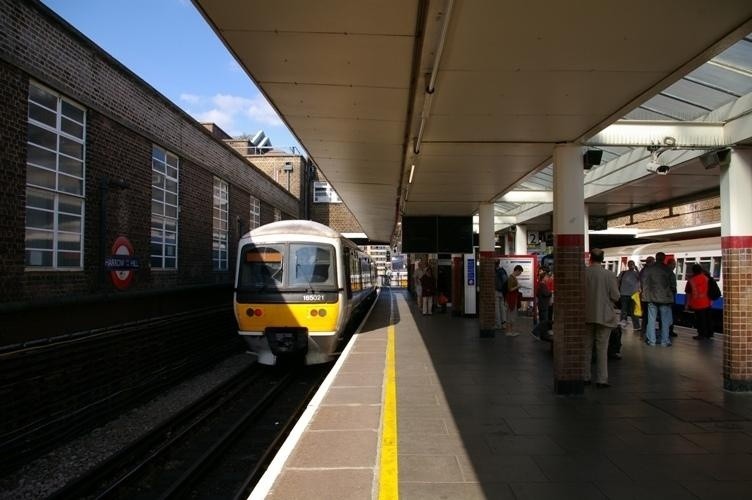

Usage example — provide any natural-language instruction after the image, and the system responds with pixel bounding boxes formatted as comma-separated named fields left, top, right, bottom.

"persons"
left=531, top=267, right=554, bottom=341
left=617, top=252, right=714, bottom=347
left=495, top=259, right=523, bottom=337
left=584, top=248, right=621, bottom=388
left=414, top=260, right=436, bottom=316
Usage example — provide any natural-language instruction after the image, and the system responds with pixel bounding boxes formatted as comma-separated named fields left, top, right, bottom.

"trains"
left=231, top=215, right=378, bottom=368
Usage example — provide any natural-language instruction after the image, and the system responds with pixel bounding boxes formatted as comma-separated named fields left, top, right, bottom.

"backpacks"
left=707, top=277, right=721, bottom=301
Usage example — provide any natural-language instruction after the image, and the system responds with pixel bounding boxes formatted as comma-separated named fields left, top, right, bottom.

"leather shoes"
left=633, top=327, right=677, bottom=346
left=585, top=380, right=609, bottom=390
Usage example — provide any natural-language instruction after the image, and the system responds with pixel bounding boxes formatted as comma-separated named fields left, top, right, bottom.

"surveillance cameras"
left=646, top=162, right=670, bottom=176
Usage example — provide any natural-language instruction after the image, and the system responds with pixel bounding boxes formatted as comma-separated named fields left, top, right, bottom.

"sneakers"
left=528, top=326, right=553, bottom=342
left=505, top=331, right=518, bottom=337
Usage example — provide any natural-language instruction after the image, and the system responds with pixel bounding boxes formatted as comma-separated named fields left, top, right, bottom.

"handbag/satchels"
left=631, top=292, right=644, bottom=317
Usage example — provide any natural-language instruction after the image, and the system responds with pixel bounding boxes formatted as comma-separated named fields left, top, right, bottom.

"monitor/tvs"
left=437, top=215, right=473, bottom=253
left=401, top=215, right=438, bottom=253
